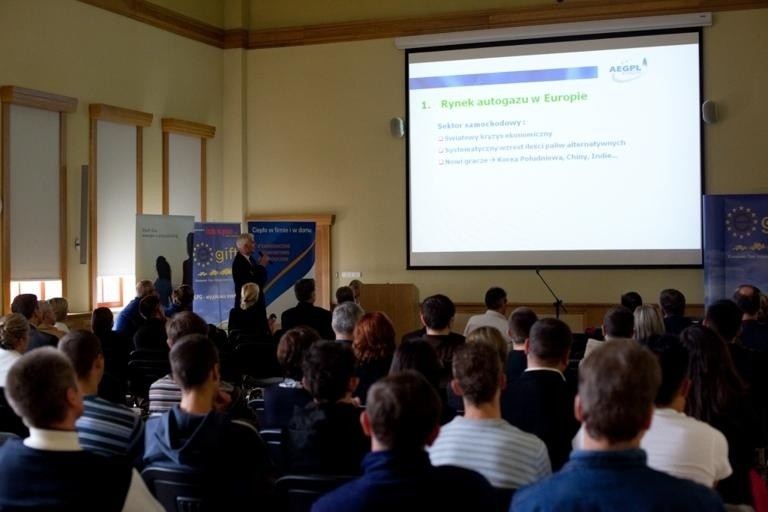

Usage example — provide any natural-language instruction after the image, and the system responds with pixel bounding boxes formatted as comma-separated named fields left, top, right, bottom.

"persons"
left=1, top=278, right=767, bottom=512
left=231, top=232, right=274, bottom=306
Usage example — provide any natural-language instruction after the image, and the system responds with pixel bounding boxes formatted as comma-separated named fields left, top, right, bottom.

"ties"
left=249, top=256, right=255, bottom=265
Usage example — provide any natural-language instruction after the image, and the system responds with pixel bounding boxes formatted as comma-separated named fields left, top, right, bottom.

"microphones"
left=257, top=248, right=271, bottom=263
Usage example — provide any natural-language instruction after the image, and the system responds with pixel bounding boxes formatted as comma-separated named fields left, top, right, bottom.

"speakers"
left=703, top=100, right=718, bottom=124
left=389, top=116, right=406, bottom=139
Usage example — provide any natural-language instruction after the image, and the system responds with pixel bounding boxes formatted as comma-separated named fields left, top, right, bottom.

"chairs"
left=141, top=465, right=362, bottom=511
left=125, top=351, right=169, bottom=404
